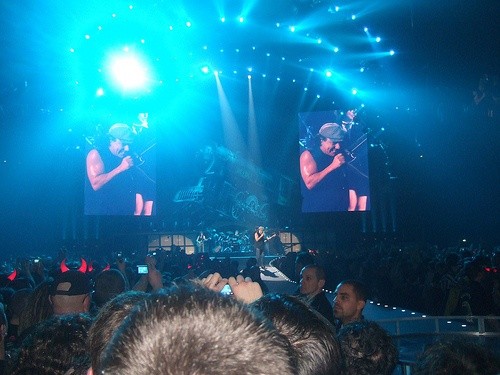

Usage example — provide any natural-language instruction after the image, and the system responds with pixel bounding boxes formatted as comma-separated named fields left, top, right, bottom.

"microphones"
left=335, top=149, right=347, bottom=176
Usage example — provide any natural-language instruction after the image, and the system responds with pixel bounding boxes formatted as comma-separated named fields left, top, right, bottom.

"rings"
left=231, top=282, right=239, bottom=289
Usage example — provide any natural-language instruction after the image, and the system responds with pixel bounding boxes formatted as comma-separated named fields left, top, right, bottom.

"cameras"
left=220, top=283, right=233, bottom=296
left=136, top=265, right=149, bottom=275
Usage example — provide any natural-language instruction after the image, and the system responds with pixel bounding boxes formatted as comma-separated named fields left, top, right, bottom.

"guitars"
left=196, top=237, right=211, bottom=247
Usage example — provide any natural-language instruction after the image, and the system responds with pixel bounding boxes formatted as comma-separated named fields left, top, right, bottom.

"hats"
left=319, top=122, right=345, bottom=142
left=49, top=271, right=91, bottom=296
left=109, top=123, right=134, bottom=145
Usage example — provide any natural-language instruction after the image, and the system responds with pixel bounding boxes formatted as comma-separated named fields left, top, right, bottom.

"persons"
left=0, top=245, right=500, bottom=375
left=83, top=112, right=155, bottom=217
left=255, top=226, right=270, bottom=270
left=299, top=122, right=345, bottom=211
left=345, top=109, right=370, bottom=212
left=196, top=231, right=208, bottom=252
